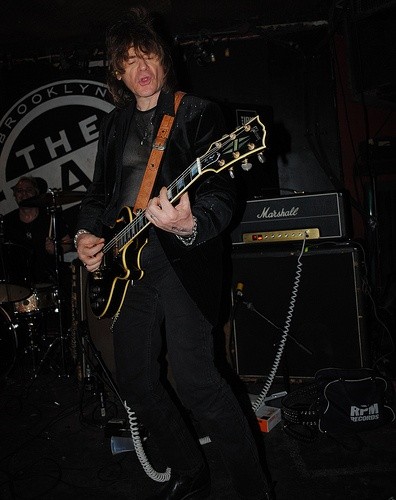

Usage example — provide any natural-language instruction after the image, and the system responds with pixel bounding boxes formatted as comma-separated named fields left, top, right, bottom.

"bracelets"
left=176, top=216, right=198, bottom=246
left=74, top=229, right=90, bottom=249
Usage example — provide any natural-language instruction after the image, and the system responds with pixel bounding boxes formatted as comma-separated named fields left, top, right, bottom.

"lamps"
left=180, top=35, right=217, bottom=71
left=76, top=27, right=106, bottom=63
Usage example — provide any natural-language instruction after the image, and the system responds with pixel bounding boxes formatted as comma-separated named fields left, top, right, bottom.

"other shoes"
left=145, top=463, right=211, bottom=500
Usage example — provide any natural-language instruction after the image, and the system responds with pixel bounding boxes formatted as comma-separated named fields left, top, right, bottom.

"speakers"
left=225, top=247, right=364, bottom=385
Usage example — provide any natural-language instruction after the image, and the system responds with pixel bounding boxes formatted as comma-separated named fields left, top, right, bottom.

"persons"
left=69, top=7, right=272, bottom=500
left=0, top=176, right=62, bottom=378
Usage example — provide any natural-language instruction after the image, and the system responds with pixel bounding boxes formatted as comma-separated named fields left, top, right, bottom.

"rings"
left=83, top=264, right=87, bottom=270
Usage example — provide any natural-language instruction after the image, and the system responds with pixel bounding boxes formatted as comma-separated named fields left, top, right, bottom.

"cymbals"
left=19, top=189, right=87, bottom=209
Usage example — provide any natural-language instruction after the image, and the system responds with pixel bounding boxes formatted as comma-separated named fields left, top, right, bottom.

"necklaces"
left=134, top=108, right=155, bottom=146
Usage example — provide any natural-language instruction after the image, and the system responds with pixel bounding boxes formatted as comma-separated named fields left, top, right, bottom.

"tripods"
left=30, top=204, right=84, bottom=382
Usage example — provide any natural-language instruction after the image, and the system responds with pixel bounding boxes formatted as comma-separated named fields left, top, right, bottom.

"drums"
left=0, top=241, right=33, bottom=303
left=0, top=305, right=28, bottom=384
left=30, top=280, right=61, bottom=308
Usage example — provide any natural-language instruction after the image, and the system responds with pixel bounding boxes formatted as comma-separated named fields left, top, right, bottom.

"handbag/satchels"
left=281, top=365, right=396, bottom=433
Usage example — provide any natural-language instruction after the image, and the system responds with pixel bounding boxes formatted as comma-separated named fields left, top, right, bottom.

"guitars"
left=86, top=115, right=268, bottom=320
